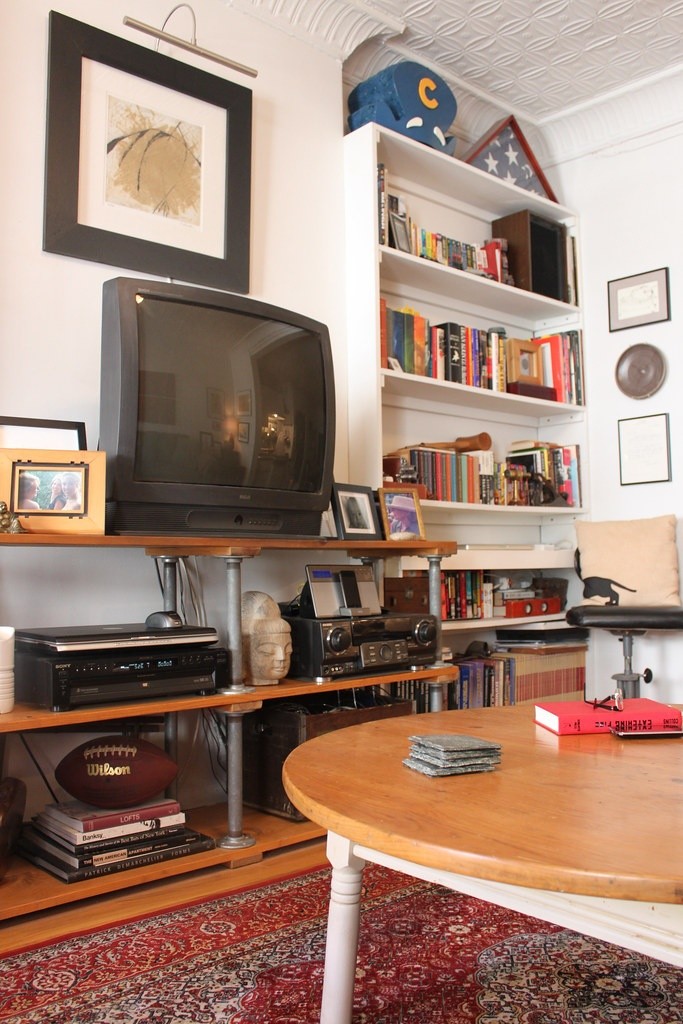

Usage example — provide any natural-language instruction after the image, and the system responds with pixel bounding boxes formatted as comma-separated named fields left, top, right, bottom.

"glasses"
left=584, top=682, right=623, bottom=712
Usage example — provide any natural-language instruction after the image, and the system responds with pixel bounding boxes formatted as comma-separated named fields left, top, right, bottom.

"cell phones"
left=338, top=570, right=362, bottom=609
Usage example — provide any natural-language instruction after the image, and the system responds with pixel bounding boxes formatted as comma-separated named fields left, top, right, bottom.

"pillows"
left=575, top=514, right=683, bottom=606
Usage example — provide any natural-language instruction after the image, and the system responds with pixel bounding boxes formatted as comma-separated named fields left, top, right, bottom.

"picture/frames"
left=238, top=422, right=249, bottom=443
left=330, top=483, right=383, bottom=540
left=390, top=211, right=412, bottom=254
left=0, top=448, right=106, bottom=535
left=213, top=442, right=224, bottom=460
left=236, top=389, right=252, bottom=417
left=199, top=432, right=214, bottom=449
left=43, top=11, right=252, bottom=295
left=617, top=414, right=673, bottom=485
left=206, top=387, right=226, bottom=420
left=607, top=267, right=671, bottom=332
left=378, top=488, right=427, bottom=541
left=0, top=416, right=87, bottom=451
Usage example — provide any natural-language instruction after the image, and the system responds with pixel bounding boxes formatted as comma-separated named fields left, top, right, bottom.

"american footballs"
left=54, top=735, right=179, bottom=810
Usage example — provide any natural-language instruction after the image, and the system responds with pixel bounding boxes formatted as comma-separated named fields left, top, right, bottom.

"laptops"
left=15, top=623, right=219, bottom=659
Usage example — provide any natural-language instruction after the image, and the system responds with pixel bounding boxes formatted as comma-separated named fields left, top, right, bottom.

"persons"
left=387, top=496, right=420, bottom=538
left=346, top=497, right=369, bottom=529
left=18, top=473, right=82, bottom=511
left=239, top=591, right=293, bottom=687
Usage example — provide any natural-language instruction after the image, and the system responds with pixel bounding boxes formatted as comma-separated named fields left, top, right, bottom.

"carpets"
left=0, top=860, right=683, bottom=1024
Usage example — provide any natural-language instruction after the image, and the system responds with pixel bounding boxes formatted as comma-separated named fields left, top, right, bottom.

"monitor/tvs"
left=96, top=276, right=335, bottom=537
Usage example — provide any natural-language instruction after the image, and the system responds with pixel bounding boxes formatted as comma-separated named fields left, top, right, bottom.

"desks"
left=283, top=703, right=683, bottom=1024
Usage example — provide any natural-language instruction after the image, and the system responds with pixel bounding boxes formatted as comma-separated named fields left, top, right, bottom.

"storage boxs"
left=492, top=210, right=571, bottom=303
left=506, top=338, right=557, bottom=401
left=226, top=695, right=417, bottom=820
left=383, top=577, right=429, bottom=615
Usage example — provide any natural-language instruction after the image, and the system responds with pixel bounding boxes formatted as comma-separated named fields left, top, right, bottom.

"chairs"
left=566, top=547, right=683, bottom=699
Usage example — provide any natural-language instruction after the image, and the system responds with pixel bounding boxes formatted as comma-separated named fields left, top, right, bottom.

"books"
left=378, top=163, right=516, bottom=287
left=379, top=298, right=584, bottom=408
left=402, top=568, right=493, bottom=618
left=381, top=440, right=582, bottom=509
left=17, top=798, right=216, bottom=886
left=532, top=698, right=683, bottom=737
left=373, top=646, right=587, bottom=717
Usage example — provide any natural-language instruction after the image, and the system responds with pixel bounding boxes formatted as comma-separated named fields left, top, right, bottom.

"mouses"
left=146, top=611, right=183, bottom=628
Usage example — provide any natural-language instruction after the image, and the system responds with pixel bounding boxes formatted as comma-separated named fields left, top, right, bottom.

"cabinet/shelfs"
left=344, top=122, right=591, bottom=631
left=0, top=532, right=458, bottom=919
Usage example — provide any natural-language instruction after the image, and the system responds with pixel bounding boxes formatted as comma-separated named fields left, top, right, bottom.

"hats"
left=385, top=496, right=416, bottom=513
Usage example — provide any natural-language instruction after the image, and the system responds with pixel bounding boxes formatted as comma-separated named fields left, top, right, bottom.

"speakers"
left=493, top=210, right=571, bottom=304
